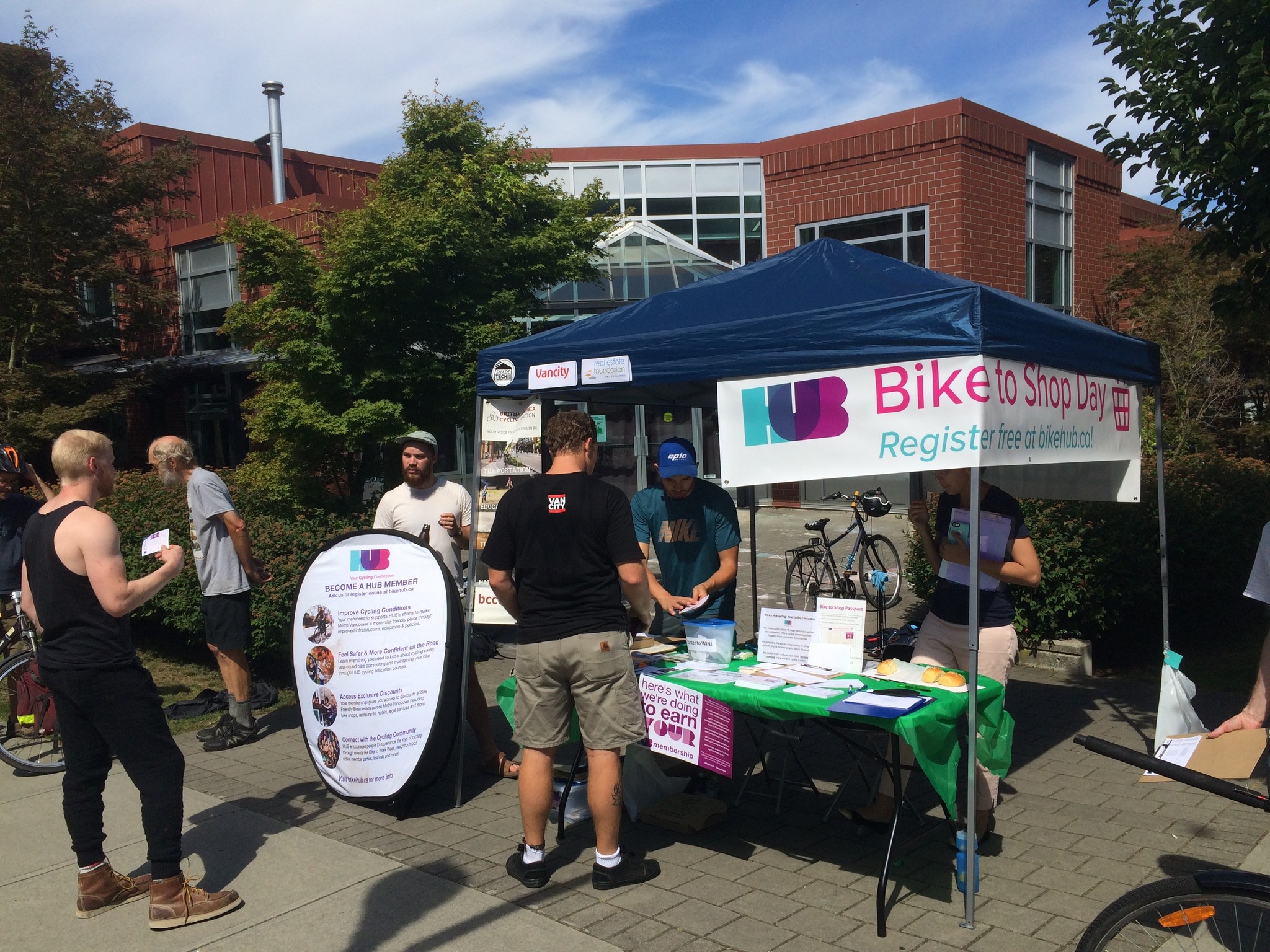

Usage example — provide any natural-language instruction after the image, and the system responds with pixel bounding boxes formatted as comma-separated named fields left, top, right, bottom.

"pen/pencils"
left=848, top=684, right=852, bottom=695
left=854, top=684, right=867, bottom=692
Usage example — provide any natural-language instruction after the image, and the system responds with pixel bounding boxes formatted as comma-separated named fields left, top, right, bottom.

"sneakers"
left=505, top=844, right=548, bottom=887
left=196, top=712, right=236, bottom=740
left=203, top=717, right=259, bottom=751
left=593, top=853, right=660, bottom=890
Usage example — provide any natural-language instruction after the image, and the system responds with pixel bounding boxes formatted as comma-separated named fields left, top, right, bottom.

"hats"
left=395, top=430, right=438, bottom=452
left=657, top=436, right=698, bottom=478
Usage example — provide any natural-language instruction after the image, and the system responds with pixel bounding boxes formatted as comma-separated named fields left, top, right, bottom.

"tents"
left=445, top=234, right=1174, bottom=932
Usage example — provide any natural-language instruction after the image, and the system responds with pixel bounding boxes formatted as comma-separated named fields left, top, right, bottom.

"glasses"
left=0, top=477, right=19, bottom=484
left=150, top=460, right=164, bottom=472
left=933, top=470, right=949, bottom=475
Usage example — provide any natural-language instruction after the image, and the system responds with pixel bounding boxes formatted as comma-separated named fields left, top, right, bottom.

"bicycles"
left=785, top=488, right=903, bottom=613
left=1, top=589, right=67, bottom=776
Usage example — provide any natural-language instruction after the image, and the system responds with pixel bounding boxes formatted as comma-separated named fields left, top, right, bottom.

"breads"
left=876, top=660, right=898, bottom=675
left=937, top=671, right=966, bottom=688
left=921, top=667, right=946, bottom=683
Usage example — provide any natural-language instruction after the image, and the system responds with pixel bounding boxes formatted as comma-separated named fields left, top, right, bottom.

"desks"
left=496, top=635, right=1016, bottom=938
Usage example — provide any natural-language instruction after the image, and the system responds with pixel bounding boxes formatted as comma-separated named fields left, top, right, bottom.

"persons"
left=0, top=442, right=56, bottom=740
left=832, top=468, right=1042, bottom=850
left=312, top=695, right=337, bottom=726
left=478, top=409, right=663, bottom=891
left=1206, top=514, right=1269, bottom=739
left=147, top=436, right=275, bottom=753
left=482, top=485, right=488, bottom=503
left=313, top=607, right=327, bottom=635
left=19, top=429, right=243, bottom=930
left=307, top=647, right=334, bottom=685
left=370, top=430, right=521, bottom=779
left=503, top=452, right=510, bottom=468
left=629, top=437, right=742, bottom=796
left=506, top=477, right=514, bottom=488
left=317, top=734, right=340, bottom=768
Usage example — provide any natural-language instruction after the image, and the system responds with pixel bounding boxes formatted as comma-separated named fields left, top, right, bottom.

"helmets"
left=863, top=496, right=892, bottom=517
left=1, top=442, right=28, bottom=473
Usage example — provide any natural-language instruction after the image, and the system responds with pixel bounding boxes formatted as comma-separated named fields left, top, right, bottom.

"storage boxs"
left=681, top=616, right=741, bottom=665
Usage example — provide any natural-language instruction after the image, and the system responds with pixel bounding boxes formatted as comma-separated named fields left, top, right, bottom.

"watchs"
left=449, top=525, right=462, bottom=538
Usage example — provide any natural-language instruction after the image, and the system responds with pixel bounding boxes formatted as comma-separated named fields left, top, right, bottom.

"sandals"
left=479, top=751, right=520, bottom=777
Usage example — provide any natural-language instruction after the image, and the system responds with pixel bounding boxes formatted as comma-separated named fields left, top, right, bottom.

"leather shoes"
left=75, top=863, right=152, bottom=918
left=148, top=872, right=241, bottom=930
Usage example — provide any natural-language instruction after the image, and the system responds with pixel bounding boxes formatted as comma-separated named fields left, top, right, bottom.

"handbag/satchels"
left=15, top=661, right=61, bottom=734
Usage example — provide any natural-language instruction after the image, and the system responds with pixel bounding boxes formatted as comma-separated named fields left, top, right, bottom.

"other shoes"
left=978, top=825, right=990, bottom=845
left=835, top=802, right=896, bottom=835
